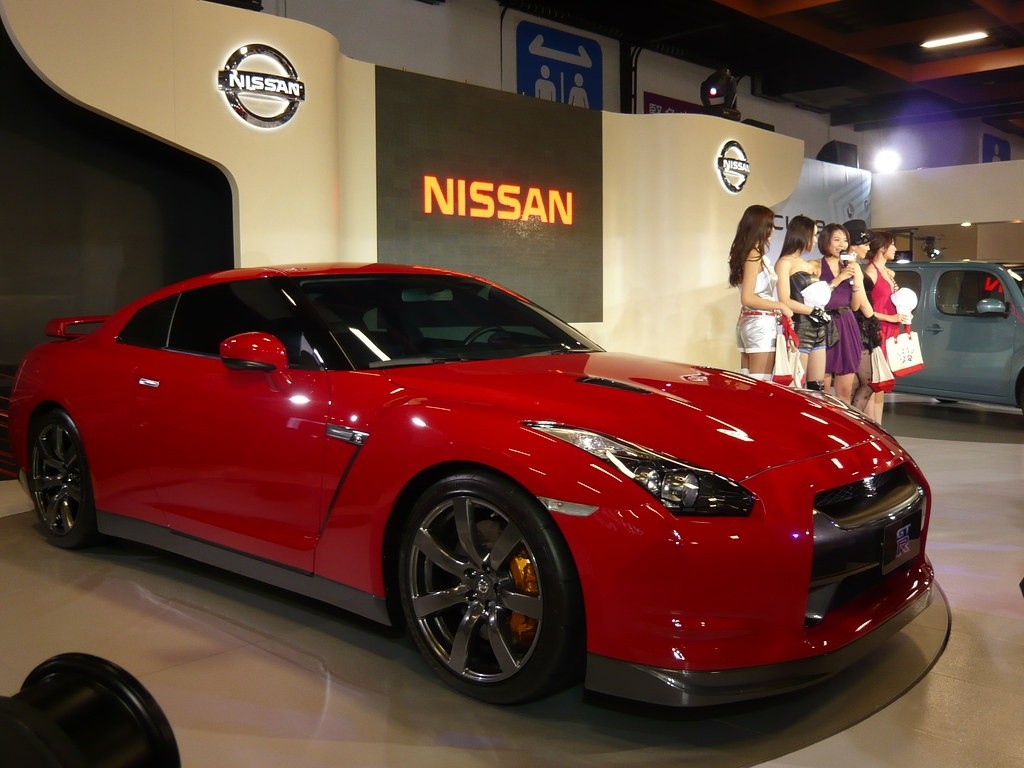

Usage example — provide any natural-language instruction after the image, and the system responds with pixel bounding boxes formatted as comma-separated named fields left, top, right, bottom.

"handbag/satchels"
left=772, top=315, right=807, bottom=390
left=883, top=323, right=924, bottom=378
left=867, top=345, right=897, bottom=394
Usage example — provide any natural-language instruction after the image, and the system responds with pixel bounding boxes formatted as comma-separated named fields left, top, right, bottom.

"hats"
left=801, top=280, right=831, bottom=311
left=843, top=219, right=874, bottom=246
left=890, top=288, right=917, bottom=325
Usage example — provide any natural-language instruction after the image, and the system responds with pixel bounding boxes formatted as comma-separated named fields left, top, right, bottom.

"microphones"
left=839, top=250, right=855, bottom=285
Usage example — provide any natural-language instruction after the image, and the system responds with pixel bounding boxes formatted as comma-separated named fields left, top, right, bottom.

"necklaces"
left=884, top=267, right=897, bottom=287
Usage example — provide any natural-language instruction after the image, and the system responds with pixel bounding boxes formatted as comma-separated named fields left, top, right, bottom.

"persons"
left=774, top=215, right=830, bottom=392
left=863, top=232, right=908, bottom=422
left=843, top=219, right=882, bottom=411
left=809, top=223, right=861, bottom=404
left=730, top=206, right=794, bottom=379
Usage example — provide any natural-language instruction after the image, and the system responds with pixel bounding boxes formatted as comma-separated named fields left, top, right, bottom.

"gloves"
left=810, top=306, right=833, bottom=328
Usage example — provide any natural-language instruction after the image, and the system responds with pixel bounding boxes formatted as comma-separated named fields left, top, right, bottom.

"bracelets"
left=829, top=282, right=836, bottom=289
left=851, top=289, right=861, bottom=293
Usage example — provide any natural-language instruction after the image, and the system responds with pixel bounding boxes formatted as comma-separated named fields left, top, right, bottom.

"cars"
left=10, top=263, right=934, bottom=710
left=804, top=263, right=1024, bottom=407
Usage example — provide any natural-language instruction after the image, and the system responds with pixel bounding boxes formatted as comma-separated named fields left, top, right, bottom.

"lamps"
left=700, top=71, right=742, bottom=121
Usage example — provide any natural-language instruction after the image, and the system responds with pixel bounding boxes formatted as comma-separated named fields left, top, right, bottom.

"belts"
left=741, top=312, right=775, bottom=316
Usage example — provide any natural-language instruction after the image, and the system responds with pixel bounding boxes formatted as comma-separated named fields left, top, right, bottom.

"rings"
left=791, top=314, right=793, bottom=316
